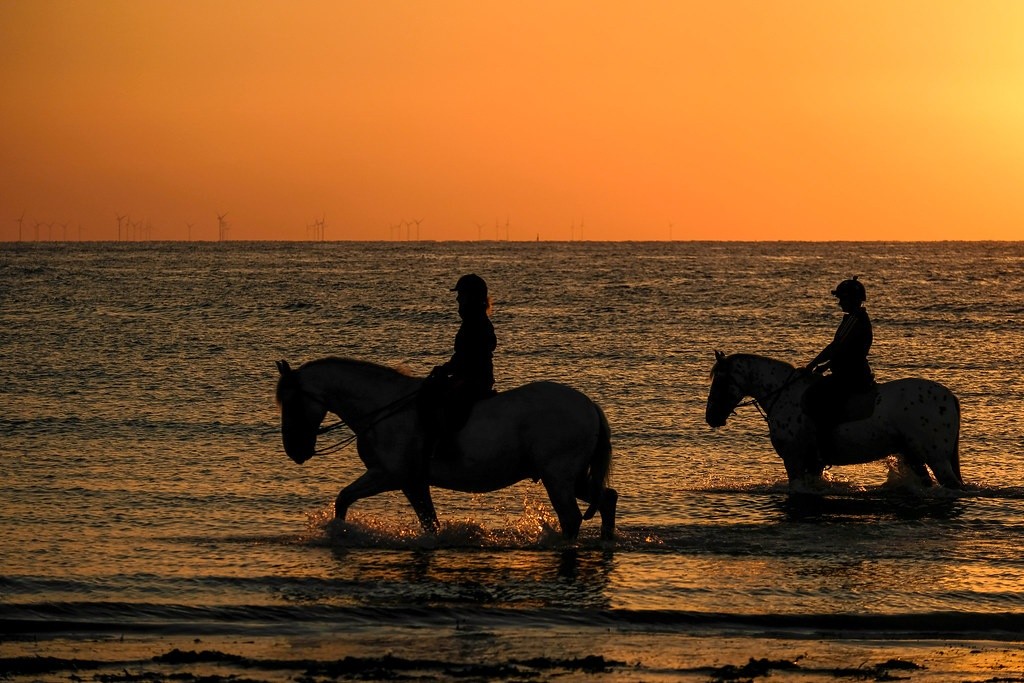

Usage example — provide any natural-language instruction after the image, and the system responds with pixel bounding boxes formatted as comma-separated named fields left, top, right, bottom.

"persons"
left=801, top=276, right=873, bottom=463
left=408, top=274, right=497, bottom=480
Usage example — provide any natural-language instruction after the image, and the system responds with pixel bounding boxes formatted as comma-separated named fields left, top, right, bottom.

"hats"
left=831, top=275, right=868, bottom=302
left=450, top=273, right=487, bottom=291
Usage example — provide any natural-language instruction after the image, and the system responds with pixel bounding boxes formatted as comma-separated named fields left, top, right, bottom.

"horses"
left=707, top=349, right=963, bottom=497
left=275, top=357, right=618, bottom=540
